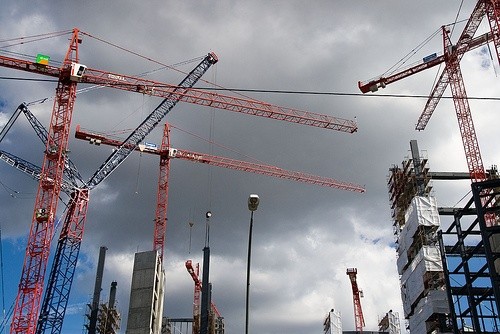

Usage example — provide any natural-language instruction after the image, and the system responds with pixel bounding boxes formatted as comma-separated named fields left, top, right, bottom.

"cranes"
left=0, top=25, right=365, bottom=333
left=359, top=1, right=499, bottom=227
left=415, top=0, right=500, bottom=132
